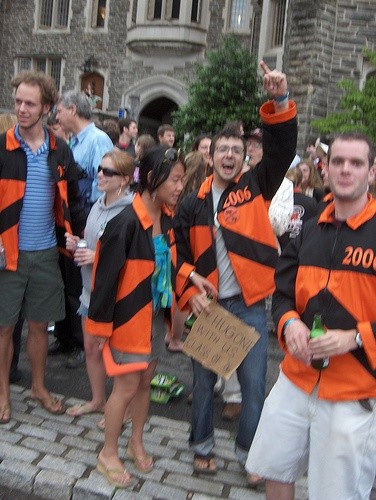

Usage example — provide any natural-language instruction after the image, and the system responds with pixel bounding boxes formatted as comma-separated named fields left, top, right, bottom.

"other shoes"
left=240, top=460, right=265, bottom=485
left=47, top=339, right=71, bottom=354
left=193, top=452, right=218, bottom=473
left=189, top=390, right=218, bottom=404
left=65, top=349, right=86, bottom=368
left=222, top=403, right=241, bottom=421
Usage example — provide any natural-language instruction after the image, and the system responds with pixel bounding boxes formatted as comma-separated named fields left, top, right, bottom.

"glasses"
left=97, top=165, right=126, bottom=177
left=218, top=145, right=245, bottom=157
left=163, top=148, right=185, bottom=164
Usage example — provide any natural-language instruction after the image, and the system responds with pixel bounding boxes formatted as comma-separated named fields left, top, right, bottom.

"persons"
left=84, top=144, right=219, bottom=488
left=244, top=129, right=376, bottom=500
left=64, top=150, right=134, bottom=430
left=172, top=58, right=298, bottom=490
left=0, top=60, right=376, bottom=500
left=0, top=70, right=73, bottom=426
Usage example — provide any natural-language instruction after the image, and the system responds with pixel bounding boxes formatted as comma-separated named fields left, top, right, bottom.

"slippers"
left=30, top=392, right=66, bottom=414
left=127, top=446, right=153, bottom=471
left=97, top=462, right=130, bottom=487
left=0, top=401, right=12, bottom=423
left=97, top=415, right=134, bottom=430
left=164, top=342, right=183, bottom=353
left=67, top=402, right=104, bottom=416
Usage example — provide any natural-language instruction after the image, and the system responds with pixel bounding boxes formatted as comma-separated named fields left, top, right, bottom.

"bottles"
left=184, top=294, right=213, bottom=329
left=310, top=313, right=329, bottom=369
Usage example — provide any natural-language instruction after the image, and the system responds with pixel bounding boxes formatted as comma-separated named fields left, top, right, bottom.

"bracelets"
left=189, top=271, right=196, bottom=278
left=274, top=98, right=289, bottom=107
left=273, top=92, right=288, bottom=101
left=283, top=318, right=296, bottom=328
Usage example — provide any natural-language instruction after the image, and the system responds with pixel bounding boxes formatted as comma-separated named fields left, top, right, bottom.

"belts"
left=360, top=398, right=374, bottom=412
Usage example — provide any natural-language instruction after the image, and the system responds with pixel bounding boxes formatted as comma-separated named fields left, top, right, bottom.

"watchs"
left=355, top=331, right=364, bottom=351
